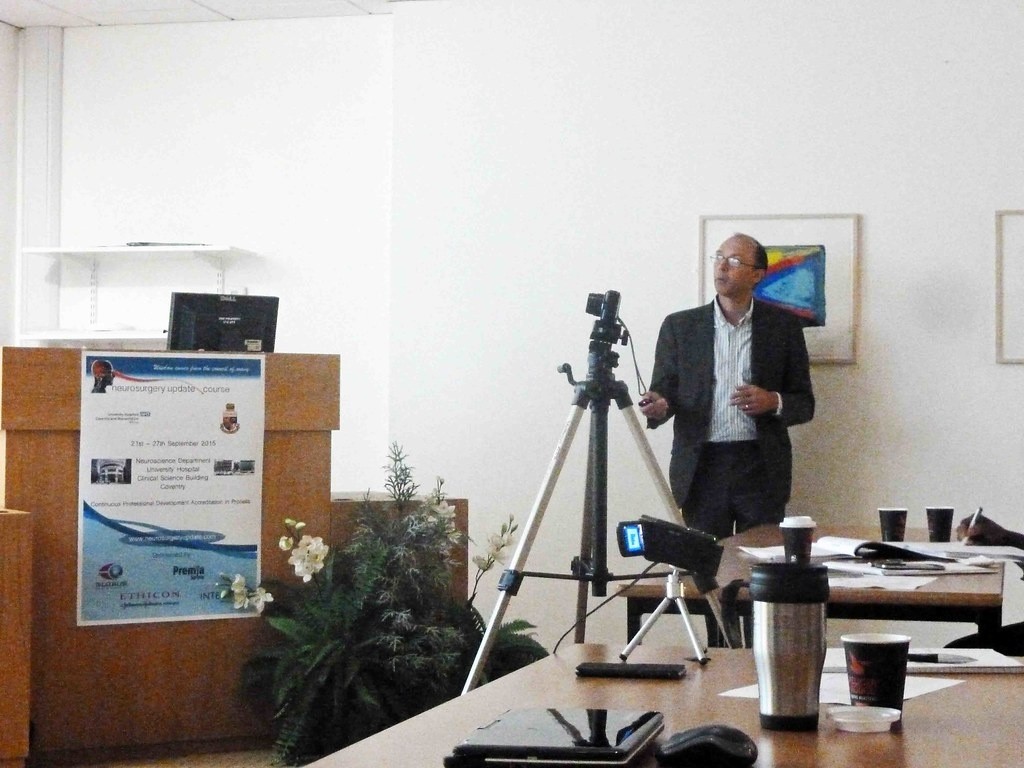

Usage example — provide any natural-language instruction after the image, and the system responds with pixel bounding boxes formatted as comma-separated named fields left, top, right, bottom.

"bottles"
left=222, top=404, right=238, bottom=430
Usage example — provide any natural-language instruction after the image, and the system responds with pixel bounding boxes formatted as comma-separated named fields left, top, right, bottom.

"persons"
left=943, top=513, right=1024, bottom=656
left=639, top=233, right=815, bottom=649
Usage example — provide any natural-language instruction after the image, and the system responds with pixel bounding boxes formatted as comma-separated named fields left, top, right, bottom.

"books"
left=823, top=647, right=1024, bottom=674
left=736, top=536, right=957, bottom=564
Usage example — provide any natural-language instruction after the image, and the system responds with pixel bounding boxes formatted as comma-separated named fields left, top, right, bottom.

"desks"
left=617, top=524, right=1005, bottom=648
left=297, top=640, right=1024, bottom=768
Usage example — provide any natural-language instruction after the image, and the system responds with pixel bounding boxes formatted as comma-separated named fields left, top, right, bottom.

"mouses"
left=655, top=725, right=758, bottom=768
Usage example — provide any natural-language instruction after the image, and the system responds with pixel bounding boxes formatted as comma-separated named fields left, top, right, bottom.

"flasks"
left=751, top=562, right=830, bottom=732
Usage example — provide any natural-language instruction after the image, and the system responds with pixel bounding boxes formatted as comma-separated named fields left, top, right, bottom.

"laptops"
left=443, top=709, right=665, bottom=768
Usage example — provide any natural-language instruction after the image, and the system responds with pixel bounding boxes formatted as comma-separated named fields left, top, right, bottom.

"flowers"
left=206, top=441, right=550, bottom=768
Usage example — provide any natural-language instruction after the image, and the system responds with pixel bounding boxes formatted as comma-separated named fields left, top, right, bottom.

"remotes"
left=638, top=398, right=653, bottom=407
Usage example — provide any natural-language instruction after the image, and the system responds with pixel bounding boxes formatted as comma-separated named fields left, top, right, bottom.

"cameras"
left=586, top=290, right=621, bottom=321
left=616, top=514, right=723, bottom=576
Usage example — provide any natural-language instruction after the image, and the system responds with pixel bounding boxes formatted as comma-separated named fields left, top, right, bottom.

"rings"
left=746, top=404, right=748, bottom=408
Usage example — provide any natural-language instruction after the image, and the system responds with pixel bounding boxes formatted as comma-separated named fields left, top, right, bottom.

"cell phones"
left=575, top=662, right=686, bottom=680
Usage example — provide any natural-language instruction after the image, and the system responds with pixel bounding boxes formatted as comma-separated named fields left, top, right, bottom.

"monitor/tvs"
left=167, top=292, right=279, bottom=353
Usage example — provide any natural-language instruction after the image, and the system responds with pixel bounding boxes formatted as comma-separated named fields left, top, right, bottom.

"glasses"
left=710, top=254, right=757, bottom=269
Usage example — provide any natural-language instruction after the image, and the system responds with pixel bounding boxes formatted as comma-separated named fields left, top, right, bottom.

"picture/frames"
left=993, top=210, right=1024, bottom=366
left=698, top=212, right=862, bottom=371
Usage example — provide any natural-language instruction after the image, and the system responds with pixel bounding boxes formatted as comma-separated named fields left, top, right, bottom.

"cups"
left=925, top=506, right=955, bottom=542
left=779, top=516, right=817, bottom=564
left=841, top=633, right=913, bottom=732
left=878, top=507, right=908, bottom=541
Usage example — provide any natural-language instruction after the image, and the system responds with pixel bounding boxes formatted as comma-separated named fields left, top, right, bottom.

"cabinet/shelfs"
left=21, top=246, right=248, bottom=340
left=330, top=490, right=470, bottom=602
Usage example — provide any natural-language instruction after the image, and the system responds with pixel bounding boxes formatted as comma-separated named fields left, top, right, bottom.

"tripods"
left=462, top=318, right=734, bottom=699
left=619, top=566, right=708, bottom=666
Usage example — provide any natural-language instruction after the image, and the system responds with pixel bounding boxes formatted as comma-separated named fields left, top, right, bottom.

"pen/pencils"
left=907, top=653, right=978, bottom=663
left=962, top=507, right=983, bottom=545
